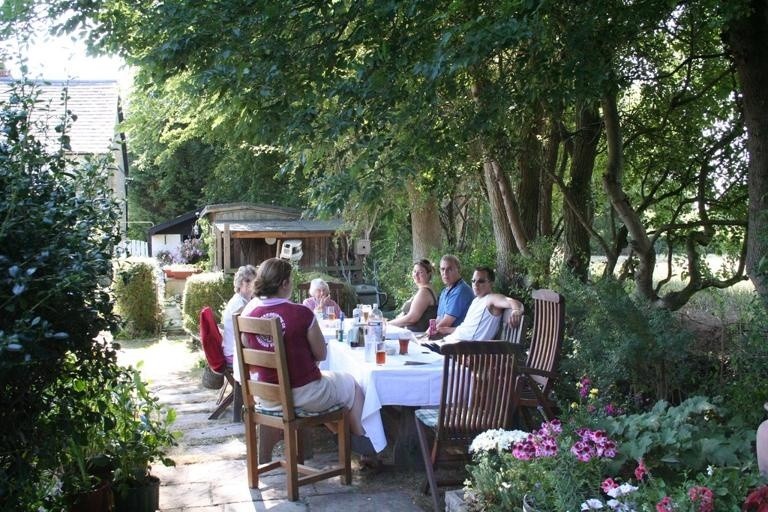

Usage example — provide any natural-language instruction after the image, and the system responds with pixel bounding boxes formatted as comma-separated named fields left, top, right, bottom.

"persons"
left=231, top=291, right=266, bottom=386
left=300, top=277, right=344, bottom=319
left=222, top=265, right=259, bottom=364
left=420, top=267, right=525, bottom=368
left=240, top=256, right=383, bottom=469
left=416, top=254, right=475, bottom=341
left=388, top=259, right=436, bottom=335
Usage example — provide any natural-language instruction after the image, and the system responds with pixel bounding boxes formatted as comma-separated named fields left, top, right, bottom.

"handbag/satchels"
left=202, top=364, right=224, bottom=389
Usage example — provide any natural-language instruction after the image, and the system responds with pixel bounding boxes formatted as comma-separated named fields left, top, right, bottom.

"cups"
left=375, top=342, right=386, bottom=366
left=398, top=335, right=410, bottom=355
left=327, top=306, right=335, bottom=324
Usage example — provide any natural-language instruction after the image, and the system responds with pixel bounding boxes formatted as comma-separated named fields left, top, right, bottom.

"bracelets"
left=511, top=309, right=520, bottom=313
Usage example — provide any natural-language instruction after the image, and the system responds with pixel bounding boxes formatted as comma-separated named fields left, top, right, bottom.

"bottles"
left=348, top=303, right=383, bottom=342
left=349, top=342, right=376, bottom=361
left=335, top=311, right=345, bottom=342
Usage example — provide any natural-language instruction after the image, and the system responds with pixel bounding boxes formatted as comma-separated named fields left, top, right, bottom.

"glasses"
left=471, top=278, right=489, bottom=283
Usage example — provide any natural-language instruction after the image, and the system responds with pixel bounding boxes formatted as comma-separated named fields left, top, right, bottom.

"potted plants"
left=30, top=351, right=187, bottom=512
